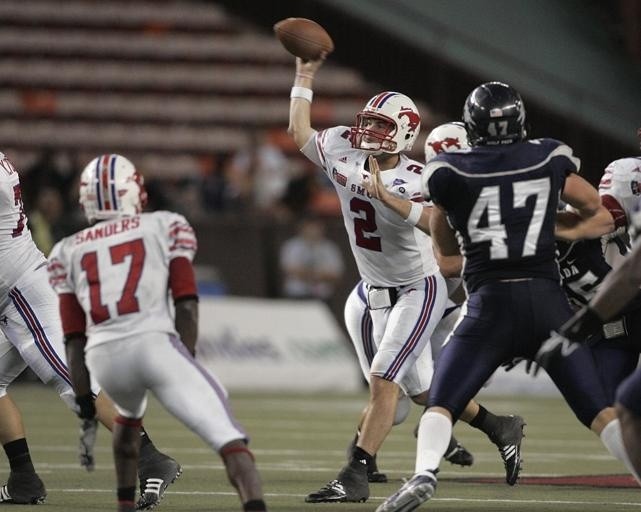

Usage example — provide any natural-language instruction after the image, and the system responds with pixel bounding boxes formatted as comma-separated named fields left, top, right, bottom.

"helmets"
left=349, top=82, right=528, bottom=163
left=76, top=154, right=149, bottom=224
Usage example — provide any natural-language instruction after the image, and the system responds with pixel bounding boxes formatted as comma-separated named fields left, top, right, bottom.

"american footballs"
left=274, top=16, right=334, bottom=61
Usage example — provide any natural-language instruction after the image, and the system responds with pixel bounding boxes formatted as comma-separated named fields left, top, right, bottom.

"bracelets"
left=295, top=70, right=316, bottom=81
left=287, top=86, right=316, bottom=104
left=73, top=392, right=97, bottom=420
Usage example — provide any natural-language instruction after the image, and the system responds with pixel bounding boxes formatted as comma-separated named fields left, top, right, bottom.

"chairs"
left=1, top=1, right=446, bottom=293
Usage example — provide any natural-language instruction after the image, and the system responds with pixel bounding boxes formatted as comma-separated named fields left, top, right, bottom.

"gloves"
left=525, top=306, right=603, bottom=378
left=79, top=418, right=98, bottom=472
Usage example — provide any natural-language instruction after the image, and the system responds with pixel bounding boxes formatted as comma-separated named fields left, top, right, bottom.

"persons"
left=282, top=50, right=527, bottom=503
left=44, top=154, right=269, bottom=511
left=341, top=79, right=640, bottom=511
left=26, top=120, right=346, bottom=302
left=1, top=152, right=185, bottom=512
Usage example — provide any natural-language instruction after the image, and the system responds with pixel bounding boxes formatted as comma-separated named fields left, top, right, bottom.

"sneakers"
left=133, top=446, right=183, bottom=511
left=0, top=473, right=47, bottom=505
left=489, top=414, right=526, bottom=486
left=413, top=425, right=473, bottom=466
left=304, top=441, right=440, bottom=512
